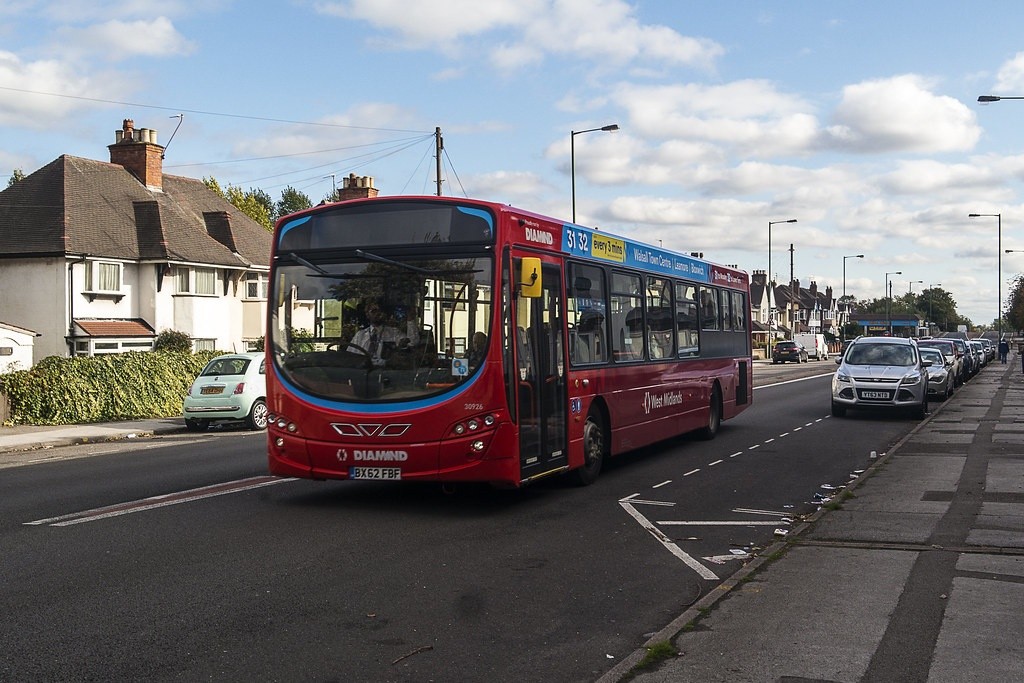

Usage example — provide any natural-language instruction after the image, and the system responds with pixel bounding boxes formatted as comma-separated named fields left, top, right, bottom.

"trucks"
left=794, top=332, right=829, bottom=361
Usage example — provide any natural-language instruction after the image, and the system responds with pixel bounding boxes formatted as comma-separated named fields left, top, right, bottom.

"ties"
left=367, top=328, right=377, bottom=357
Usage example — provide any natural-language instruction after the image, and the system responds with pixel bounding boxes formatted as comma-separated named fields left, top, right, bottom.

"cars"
left=918, top=347, right=955, bottom=401
left=182, top=351, right=285, bottom=433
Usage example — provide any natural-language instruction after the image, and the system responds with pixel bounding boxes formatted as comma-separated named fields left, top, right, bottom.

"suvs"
left=831, top=334, right=938, bottom=420
left=772, top=340, right=808, bottom=364
left=914, top=334, right=997, bottom=389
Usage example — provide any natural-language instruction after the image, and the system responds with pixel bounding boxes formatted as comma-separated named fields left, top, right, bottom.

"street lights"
left=929, top=284, right=942, bottom=335
left=909, top=280, right=923, bottom=338
left=886, top=271, right=902, bottom=331
left=969, top=214, right=1002, bottom=361
left=768, top=219, right=799, bottom=360
left=571, top=124, right=620, bottom=329
left=843, top=255, right=864, bottom=340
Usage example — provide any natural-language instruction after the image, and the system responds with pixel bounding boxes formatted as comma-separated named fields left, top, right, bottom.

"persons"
left=347, top=298, right=420, bottom=366
left=900, top=329, right=911, bottom=338
left=463, top=331, right=487, bottom=378
left=998, top=338, right=1010, bottom=364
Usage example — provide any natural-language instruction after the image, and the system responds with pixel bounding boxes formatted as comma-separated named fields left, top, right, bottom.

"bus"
left=265, top=194, right=755, bottom=493
